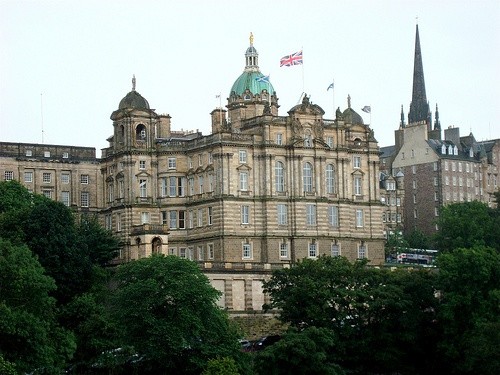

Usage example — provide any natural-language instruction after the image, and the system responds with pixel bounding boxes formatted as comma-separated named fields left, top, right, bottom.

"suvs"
left=237, top=335, right=280, bottom=351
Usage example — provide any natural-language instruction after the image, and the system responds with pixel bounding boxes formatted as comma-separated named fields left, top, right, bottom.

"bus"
left=397, top=253, right=432, bottom=265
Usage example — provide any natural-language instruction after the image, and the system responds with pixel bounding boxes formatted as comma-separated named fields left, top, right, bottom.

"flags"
left=362, top=106, right=370, bottom=113
left=280, top=49, right=303, bottom=67
left=216, top=95, right=220, bottom=98
left=327, top=83, right=333, bottom=91
